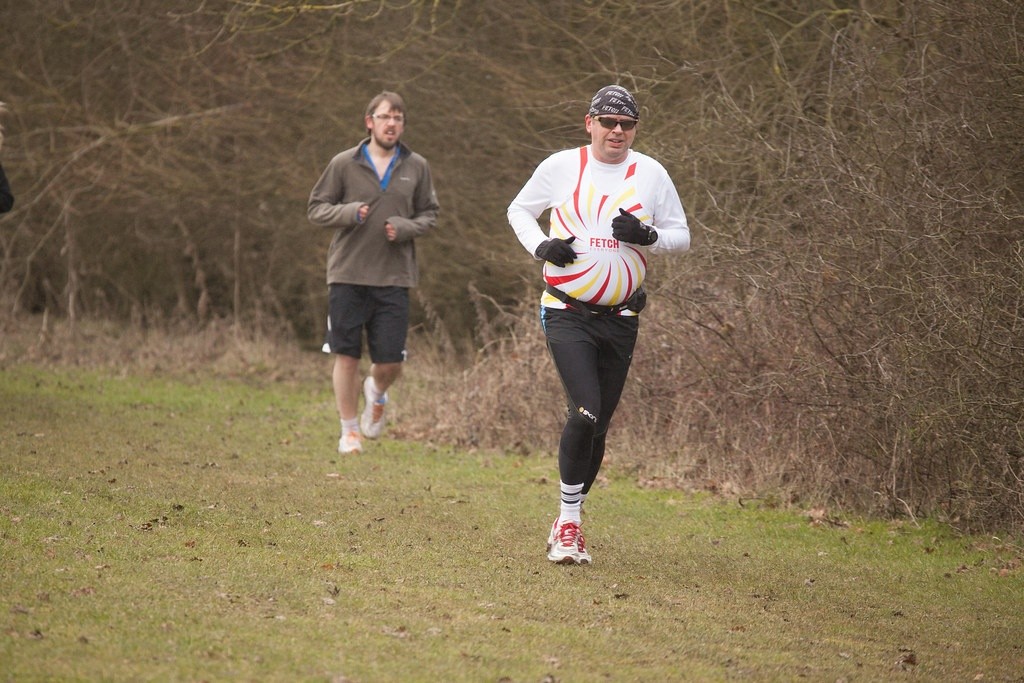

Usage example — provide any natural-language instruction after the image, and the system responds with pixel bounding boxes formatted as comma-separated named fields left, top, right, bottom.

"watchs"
left=640, top=226, right=658, bottom=246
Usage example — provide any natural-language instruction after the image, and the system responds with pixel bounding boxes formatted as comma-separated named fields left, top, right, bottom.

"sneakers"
left=338, top=430, right=364, bottom=456
left=361, top=376, right=388, bottom=440
left=576, top=531, right=593, bottom=566
left=548, top=517, right=581, bottom=565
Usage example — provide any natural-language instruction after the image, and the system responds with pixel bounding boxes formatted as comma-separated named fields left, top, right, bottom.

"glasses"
left=369, top=112, right=406, bottom=124
left=592, top=115, right=639, bottom=131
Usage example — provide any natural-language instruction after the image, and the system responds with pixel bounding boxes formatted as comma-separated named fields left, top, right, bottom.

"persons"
left=507, top=84, right=690, bottom=560
left=307, top=92, right=440, bottom=454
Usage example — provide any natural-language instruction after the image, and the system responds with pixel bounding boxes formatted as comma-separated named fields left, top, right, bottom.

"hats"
left=588, top=85, right=640, bottom=120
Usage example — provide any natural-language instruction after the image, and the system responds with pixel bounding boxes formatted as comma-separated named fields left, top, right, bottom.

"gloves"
left=611, top=207, right=652, bottom=246
left=535, top=235, right=578, bottom=268
